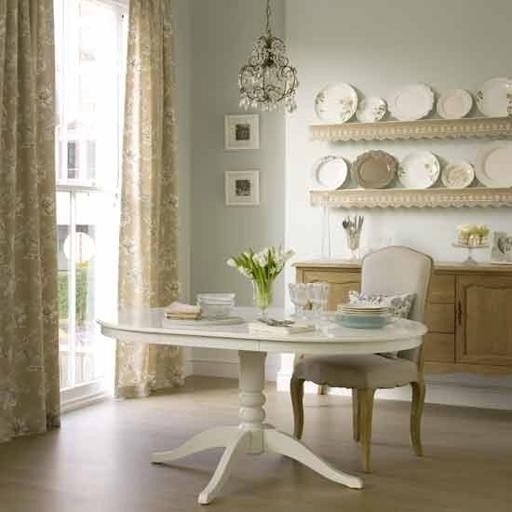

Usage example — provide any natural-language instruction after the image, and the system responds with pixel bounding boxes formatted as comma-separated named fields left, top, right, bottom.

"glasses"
left=235, top=1, right=299, bottom=115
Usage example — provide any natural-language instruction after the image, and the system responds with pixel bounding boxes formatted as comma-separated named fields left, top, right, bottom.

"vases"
left=226, top=245, right=299, bottom=301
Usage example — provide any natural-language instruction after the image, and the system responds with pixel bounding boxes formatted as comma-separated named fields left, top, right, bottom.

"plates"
left=293, top=263, right=456, bottom=376
left=455, top=266, right=512, bottom=374
left=308, top=118, right=512, bottom=210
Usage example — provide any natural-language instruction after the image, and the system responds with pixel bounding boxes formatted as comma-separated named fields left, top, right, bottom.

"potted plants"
left=222, top=169, right=261, bottom=206
left=221, top=113, right=261, bottom=152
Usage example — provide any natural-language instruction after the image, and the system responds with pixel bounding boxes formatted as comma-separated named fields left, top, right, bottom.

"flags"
left=252, top=278, right=272, bottom=319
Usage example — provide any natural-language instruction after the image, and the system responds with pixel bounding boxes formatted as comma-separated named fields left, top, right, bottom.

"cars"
left=347, top=227, right=360, bottom=251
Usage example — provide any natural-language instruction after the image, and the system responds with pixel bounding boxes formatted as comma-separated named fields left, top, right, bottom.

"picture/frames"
left=95, top=305, right=426, bottom=506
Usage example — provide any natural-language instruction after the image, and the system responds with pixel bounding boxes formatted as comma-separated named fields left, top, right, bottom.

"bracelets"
left=195, top=292, right=235, bottom=320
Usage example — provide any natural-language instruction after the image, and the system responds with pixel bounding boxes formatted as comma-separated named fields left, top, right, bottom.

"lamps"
left=288, top=244, right=435, bottom=474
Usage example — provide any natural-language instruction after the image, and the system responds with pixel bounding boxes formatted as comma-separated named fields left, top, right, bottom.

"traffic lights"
left=348, top=289, right=417, bottom=359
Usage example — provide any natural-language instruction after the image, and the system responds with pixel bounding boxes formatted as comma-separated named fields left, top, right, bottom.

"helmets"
left=313, top=140, right=512, bottom=191
left=335, top=304, right=388, bottom=319
left=315, top=77, right=512, bottom=125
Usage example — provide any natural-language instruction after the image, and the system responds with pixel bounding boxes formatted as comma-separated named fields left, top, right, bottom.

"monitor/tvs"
left=164, top=301, right=200, bottom=320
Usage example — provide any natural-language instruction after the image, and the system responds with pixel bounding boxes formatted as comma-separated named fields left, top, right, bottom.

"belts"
left=287, top=282, right=328, bottom=323
